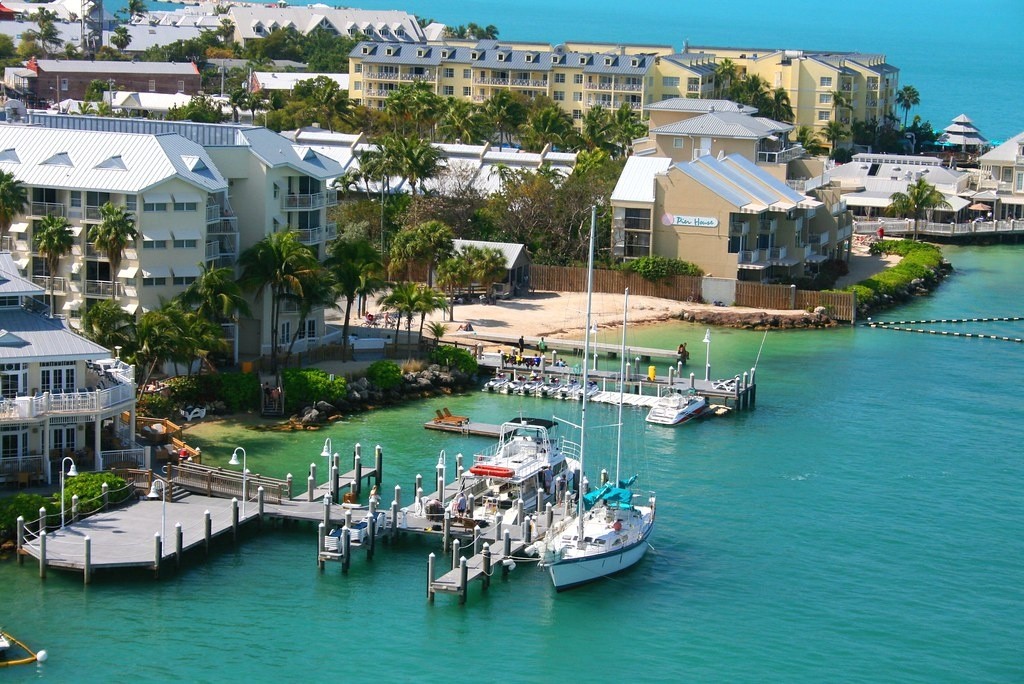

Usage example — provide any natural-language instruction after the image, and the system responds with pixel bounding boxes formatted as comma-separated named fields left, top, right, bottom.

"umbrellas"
left=968, top=203, right=991, bottom=218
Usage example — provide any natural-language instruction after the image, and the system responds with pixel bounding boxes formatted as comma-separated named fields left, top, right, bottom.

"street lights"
left=321, top=438, right=333, bottom=506
left=228, top=447, right=247, bottom=518
left=146, top=479, right=167, bottom=557
left=59, top=457, right=79, bottom=529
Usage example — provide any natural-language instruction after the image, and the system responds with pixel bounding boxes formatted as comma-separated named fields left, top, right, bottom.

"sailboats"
left=538, top=205, right=657, bottom=591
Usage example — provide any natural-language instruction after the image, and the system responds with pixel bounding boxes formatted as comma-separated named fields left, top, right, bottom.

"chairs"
left=141, top=424, right=167, bottom=444
left=64, top=446, right=93, bottom=466
left=102, top=430, right=129, bottom=450
left=5, top=467, right=39, bottom=489
left=712, top=379, right=742, bottom=392
left=433, top=408, right=469, bottom=426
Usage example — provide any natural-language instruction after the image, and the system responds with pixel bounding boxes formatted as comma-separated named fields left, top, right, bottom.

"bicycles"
left=361, top=321, right=378, bottom=328
left=382, top=322, right=396, bottom=329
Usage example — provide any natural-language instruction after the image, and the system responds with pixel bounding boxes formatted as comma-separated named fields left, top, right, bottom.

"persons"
left=497, top=335, right=567, bottom=367
left=489, top=286, right=497, bottom=304
left=975, top=149, right=978, bottom=156
left=349, top=334, right=357, bottom=348
left=363, top=311, right=415, bottom=327
left=877, top=227, right=884, bottom=239
left=541, top=465, right=552, bottom=495
left=457, top=493, right=466, bottom=518
left=507, top=490, right=520, bottom=500
left=556, top=475, right=569, bottom=506
left=851, top=234, right=874, bottom=246
left=944, top=211, right=1013, bottom=224
left=613, top=519, right=622, bottom=533
left=456, top=322, right=469, bottom=332
left=143, top=381, right=167, bottom=396
left=264, top=382, right=280, bottom=412
left=429, top=499, right=442, bottom=522
left=523, top=275, right=535, bottom=294
left=678, top=343, right=690, bottom=360
left=805, top=263, right=810, bottom=276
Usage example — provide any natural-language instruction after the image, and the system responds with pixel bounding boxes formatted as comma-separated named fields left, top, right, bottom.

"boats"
left=400, top=421, right=585, bottom=526
left=644, top=389, right=707, bottom=426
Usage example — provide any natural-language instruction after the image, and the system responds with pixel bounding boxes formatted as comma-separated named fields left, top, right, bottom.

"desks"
left=75, top=449, right=87, bottom=458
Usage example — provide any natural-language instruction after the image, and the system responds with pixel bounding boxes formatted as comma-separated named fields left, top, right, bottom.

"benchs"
left=155, top=445, right=180, bottom=462
left=107, top=460, right=138, bottom=470
